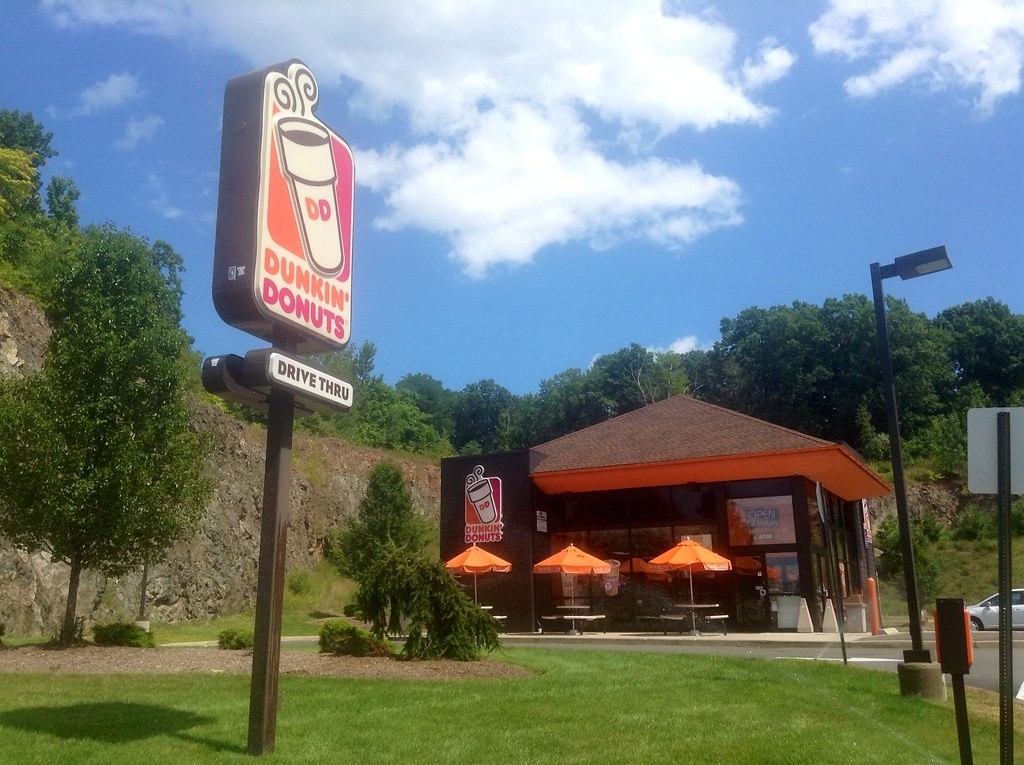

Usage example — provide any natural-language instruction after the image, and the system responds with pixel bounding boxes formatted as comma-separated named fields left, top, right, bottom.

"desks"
left=555, top=605, right=590, bottom=635
left=481, top=603, right=493, bottom=612
left=675, top=601, right=720, bottom=636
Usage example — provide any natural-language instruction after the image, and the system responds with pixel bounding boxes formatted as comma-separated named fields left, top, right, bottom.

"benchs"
left=492, top=615, right=508, bottom=635
left=661, top=614, right=686, bottom=635
left=564, top=614, right=606, bottom=634
left=704, top=614, right=730, bottom=636
left=536, top=615, right=564, bottom=636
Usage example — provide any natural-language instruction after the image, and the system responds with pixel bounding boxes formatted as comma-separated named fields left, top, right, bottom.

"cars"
left=965, top=588, right=1024, bottom=632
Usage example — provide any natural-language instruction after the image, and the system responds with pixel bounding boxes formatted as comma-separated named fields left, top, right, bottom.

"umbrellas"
left=647, top=536, right=732, bottom=629
left=618, top=558, right=672, bottom=582
left=442, top=543, right=512, bottom=607
left=533, top=543, right=611, bottom=632
left=728, top=555, right=780, bottom=579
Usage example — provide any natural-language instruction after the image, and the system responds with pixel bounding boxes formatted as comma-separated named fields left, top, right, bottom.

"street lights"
left=869, top=245, right=954, bottom=702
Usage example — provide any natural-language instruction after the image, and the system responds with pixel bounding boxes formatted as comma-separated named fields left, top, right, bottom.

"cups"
left=603, top=559, right=620, bottom=596
left=561, top=571, right=578, bottom=596
